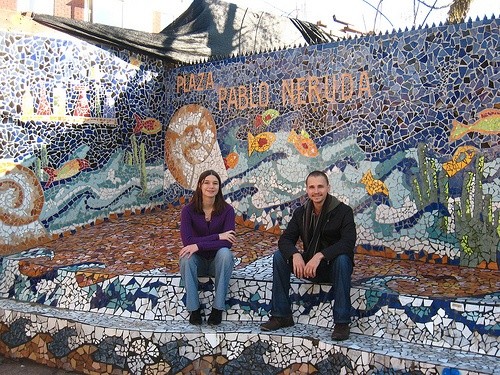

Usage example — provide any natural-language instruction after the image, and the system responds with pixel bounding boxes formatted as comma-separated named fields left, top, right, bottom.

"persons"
left=180, top=169, right=236, bottom=326
left=259, top=170, right=357, bottom=340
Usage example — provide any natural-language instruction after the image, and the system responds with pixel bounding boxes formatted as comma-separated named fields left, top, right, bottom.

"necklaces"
left=205, top=212, right=211, bottom=220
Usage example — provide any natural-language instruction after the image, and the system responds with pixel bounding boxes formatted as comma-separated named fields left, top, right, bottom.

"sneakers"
left=331, top=323, right=350, bottom=341
left=260, top=316, right=294, bottom=330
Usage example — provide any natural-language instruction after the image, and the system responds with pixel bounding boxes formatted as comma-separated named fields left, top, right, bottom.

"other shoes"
left=189, top=311, right=202, bottom=325
left=207, top=312, right=222, bottom=326
